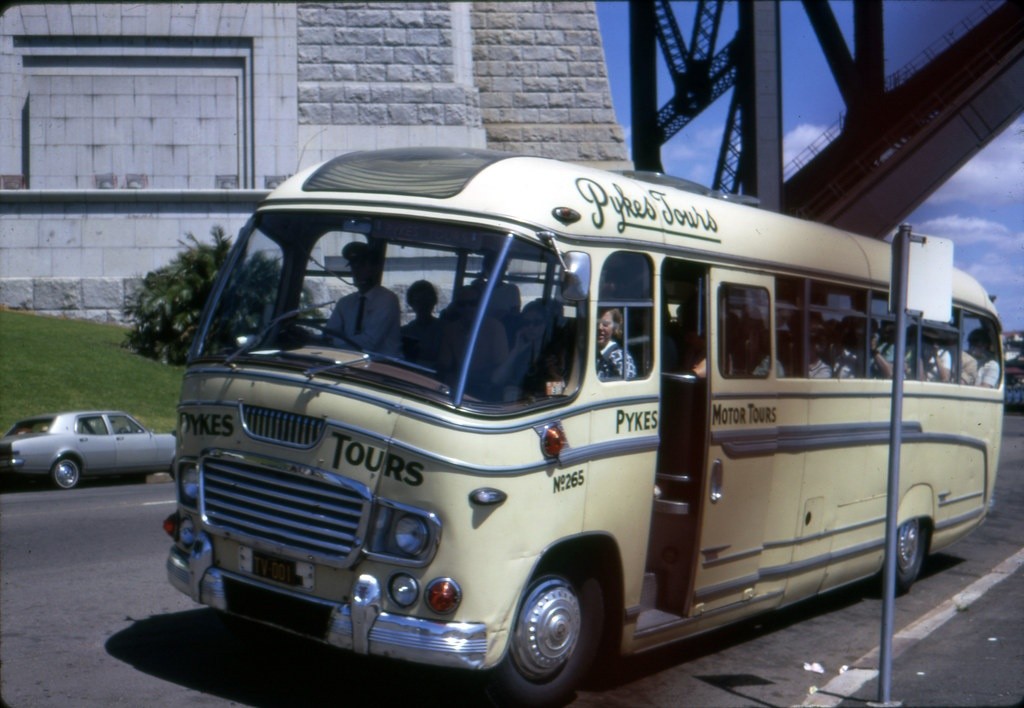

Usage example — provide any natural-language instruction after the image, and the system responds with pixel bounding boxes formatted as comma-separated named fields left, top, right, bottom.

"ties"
left=355, top=296, right=366, bottom=335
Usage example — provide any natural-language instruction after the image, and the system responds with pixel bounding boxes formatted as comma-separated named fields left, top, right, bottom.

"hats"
left=343, top=242, right=383, bottom=261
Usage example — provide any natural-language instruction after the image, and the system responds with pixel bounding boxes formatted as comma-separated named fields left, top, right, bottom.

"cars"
left=0, top=403, right=181, bottom=486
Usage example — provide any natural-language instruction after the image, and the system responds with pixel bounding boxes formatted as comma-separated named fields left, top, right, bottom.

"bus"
left=160, top=150, right=1009, bottom=708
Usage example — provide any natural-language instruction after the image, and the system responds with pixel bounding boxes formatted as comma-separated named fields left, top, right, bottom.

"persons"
left=398, top=279, right=456, bottom=373
left=597, top=307, right=638, bottom=380
left=457, top=273, right=1001, bottom=401
left=322, top=244, right=405, bottom=360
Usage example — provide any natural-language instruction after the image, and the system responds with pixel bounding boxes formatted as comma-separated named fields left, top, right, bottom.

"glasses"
left=457, top=298, right=479, bottom=307
left=597, top=319, right=615, bottom=327
